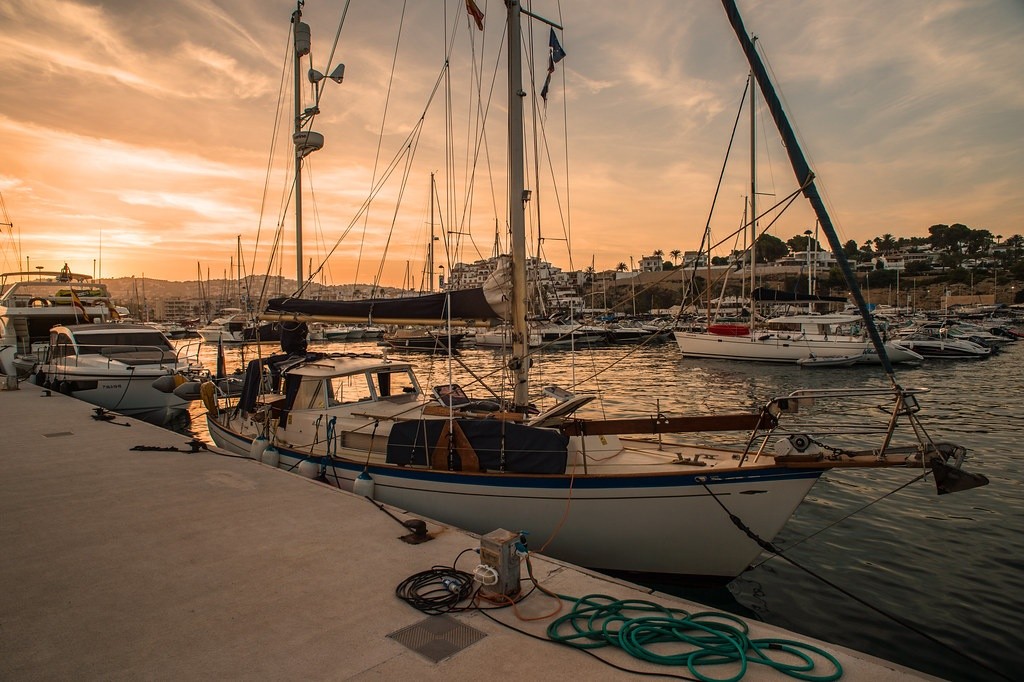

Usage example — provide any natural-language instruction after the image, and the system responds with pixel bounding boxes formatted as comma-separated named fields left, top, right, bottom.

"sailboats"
left=1, top=1, right=1024, bottom=428
left=201, top=2, right=990, bottom=591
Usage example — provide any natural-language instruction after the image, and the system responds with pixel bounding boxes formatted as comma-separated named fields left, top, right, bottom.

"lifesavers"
left=28, top=296, right=48, bottom=307
